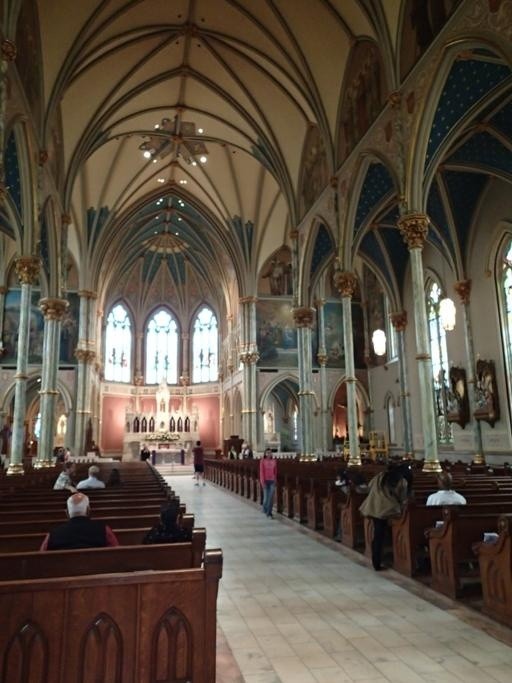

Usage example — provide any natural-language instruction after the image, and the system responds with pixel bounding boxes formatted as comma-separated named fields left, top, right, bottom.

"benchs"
left=204, top=454, right=512, bottom=630
left=0, top=455, right=223, bottom=683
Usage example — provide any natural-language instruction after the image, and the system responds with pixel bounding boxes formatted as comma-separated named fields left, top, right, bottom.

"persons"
left=64, top=447, right=71, bottom=464
left=40, top=491, right=120, bottom=552
left=192, top=440, right=206, bottom=486
left=141, top=502, right=191, bottom=545
left=358, top=463, right=416, bottom=571
left=426, top=471, right=467, bottom=507
left=259, top=448, right=278, bottom=520
left=241, top=444, right=253, bottom=460
left=335, top=475, right=347, bottom=495
left=141, top=444, right=150, bottom=461
left=53, top=462, right=124, bottom=493
left=228, top=445, right=239, bottom=460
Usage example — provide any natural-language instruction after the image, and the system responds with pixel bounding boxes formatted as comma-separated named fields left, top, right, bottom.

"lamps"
left=438, top=181, right=457, bottom=330
left=371, top=230, right=387, bottom=356
